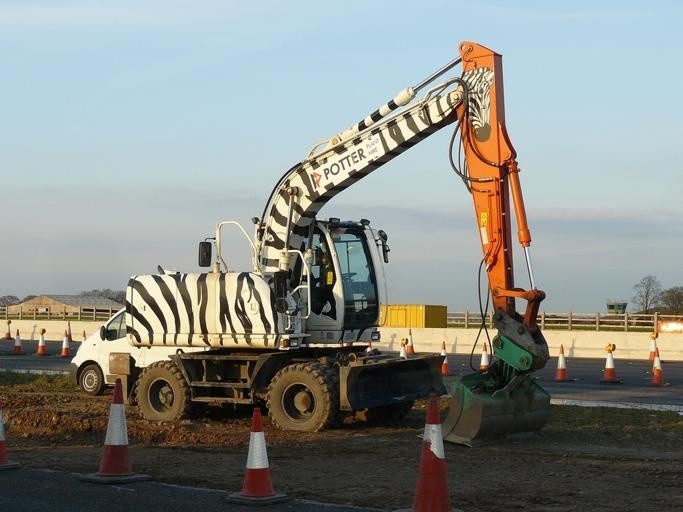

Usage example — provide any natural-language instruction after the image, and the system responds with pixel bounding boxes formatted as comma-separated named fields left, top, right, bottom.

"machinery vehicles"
left=125, top=41, right=551, bottom=449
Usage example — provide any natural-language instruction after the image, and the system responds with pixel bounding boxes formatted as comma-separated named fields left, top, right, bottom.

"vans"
left=70, top=306, right=211, bottom=397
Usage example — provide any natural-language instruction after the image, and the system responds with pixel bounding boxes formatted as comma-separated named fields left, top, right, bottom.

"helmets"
left=319, top=255, right=329, bottom=265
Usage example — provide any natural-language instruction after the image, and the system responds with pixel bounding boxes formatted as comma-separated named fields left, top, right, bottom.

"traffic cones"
left=650, top=338, right=664, bottom=385
left=410, top=392, right=452, bottom=511
left=241, top=407, right=276, bottom=497
left=98, top=379, right=133, bottom=476
left=0, top=398, right=8, bottom=464
left=603, top=348, right=619, bottom=383
left=556, top=345, right=569, bottom=380
left=5, top=320, right=86, bottom=358
left=399, top=329, right=490, bottom=376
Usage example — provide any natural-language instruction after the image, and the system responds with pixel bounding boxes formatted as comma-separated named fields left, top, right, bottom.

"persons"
left=314, top=233, right=337, bottom=320
